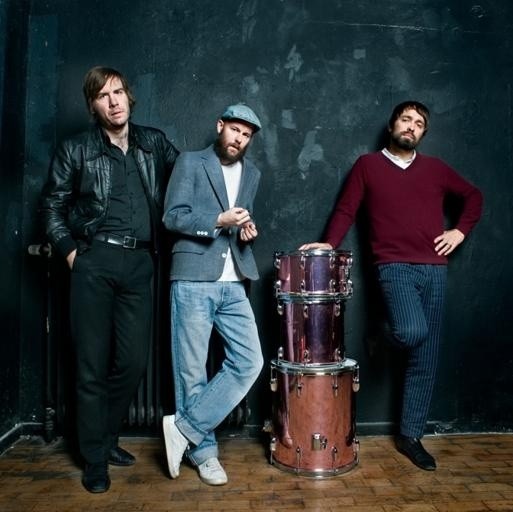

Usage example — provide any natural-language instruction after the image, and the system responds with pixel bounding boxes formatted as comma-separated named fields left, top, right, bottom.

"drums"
left=277, top=300, right=346, bottom=365
left=273, top=249, right=354, bottom=299
left=263, top=358, right=360, bottom=480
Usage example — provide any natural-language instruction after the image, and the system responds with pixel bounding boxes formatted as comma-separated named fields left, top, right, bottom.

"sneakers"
left=162, top=414, right=190, bottom=479
left=199, top=456, right=228, bottom=486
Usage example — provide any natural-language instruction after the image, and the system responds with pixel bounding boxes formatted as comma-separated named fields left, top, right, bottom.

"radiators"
left=28, top=242, right=253, bottom=442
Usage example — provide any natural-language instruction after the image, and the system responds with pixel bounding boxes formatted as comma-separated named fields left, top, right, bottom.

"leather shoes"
left=81, top=460, right=111, bottom=493
left=110, top=445, right=138, bottom=466
left=394, top=432, right=436, bottom=471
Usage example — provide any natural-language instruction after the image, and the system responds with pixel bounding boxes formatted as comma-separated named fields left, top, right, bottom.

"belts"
left=94, top=231, right=155, bottom=250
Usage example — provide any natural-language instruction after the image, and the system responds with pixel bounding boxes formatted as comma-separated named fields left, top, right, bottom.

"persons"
left=162, top=104, right=265, bottom=486
left=43, top=65, right=181, bottom=494
left=297, top=100, right=484, bottom=471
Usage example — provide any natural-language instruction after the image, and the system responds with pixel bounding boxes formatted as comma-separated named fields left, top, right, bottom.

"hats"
left=223, top=103, right=261, bottom=131
